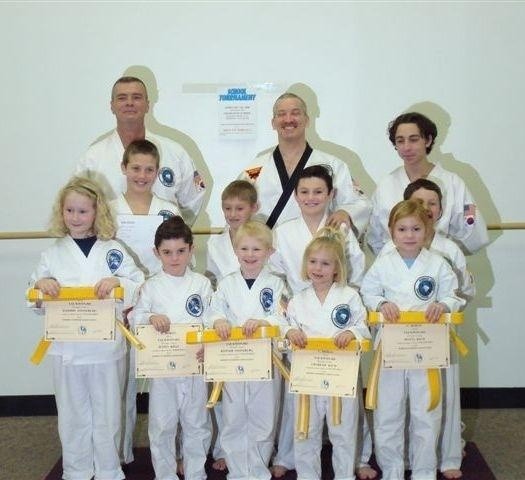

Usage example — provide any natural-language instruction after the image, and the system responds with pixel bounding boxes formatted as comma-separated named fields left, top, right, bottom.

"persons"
left=236, top=94, right=373, bottom=240
left=108, top=138, right=197, bottom=475
left=24, top=179, right=145, bottom=480
left=361, top=179, right=476, bottom=479
left=205, top=178, right=292, bottom=480
left=133, top=216, right=213, bottom=480
left=365, top=112, right=488, bottom=254
left=76, top=77, right=206, bottom=227
left=269, top=165, right=377, bottom=479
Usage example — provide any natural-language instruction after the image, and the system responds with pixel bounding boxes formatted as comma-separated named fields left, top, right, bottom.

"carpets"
left=44, top=442, right=498, bottom=479
